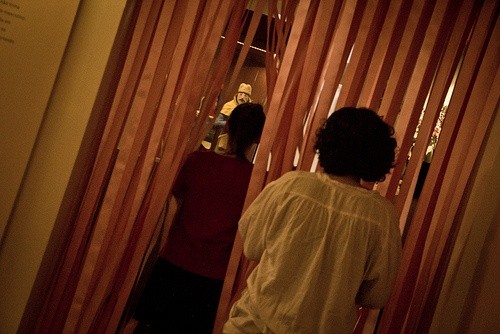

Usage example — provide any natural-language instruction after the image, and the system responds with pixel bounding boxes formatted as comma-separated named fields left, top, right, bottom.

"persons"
left=125, top=101, right=272, bottom=334
left=212, top=83, right=255, bottom=153
left=220, top=105, right=403, bottom=334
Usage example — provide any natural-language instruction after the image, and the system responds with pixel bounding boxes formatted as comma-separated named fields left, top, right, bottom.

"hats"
left=238, top=82, right=251, bottom=97
